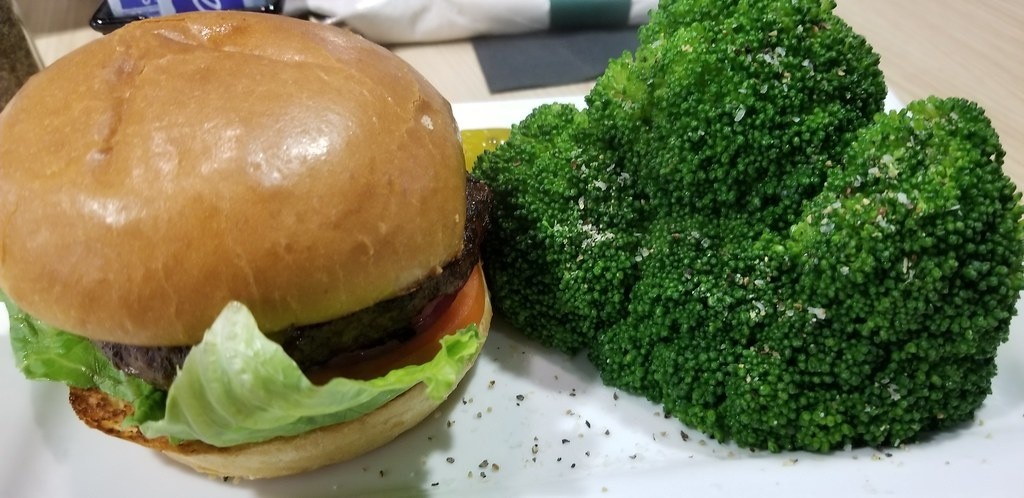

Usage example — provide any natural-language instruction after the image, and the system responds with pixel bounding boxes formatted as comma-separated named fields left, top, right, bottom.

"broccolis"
left=464, top=1, right=1024, bottom=452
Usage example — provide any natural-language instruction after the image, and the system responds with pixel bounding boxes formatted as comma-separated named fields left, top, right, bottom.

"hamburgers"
left=0, top=11, right=490, bottom=478
